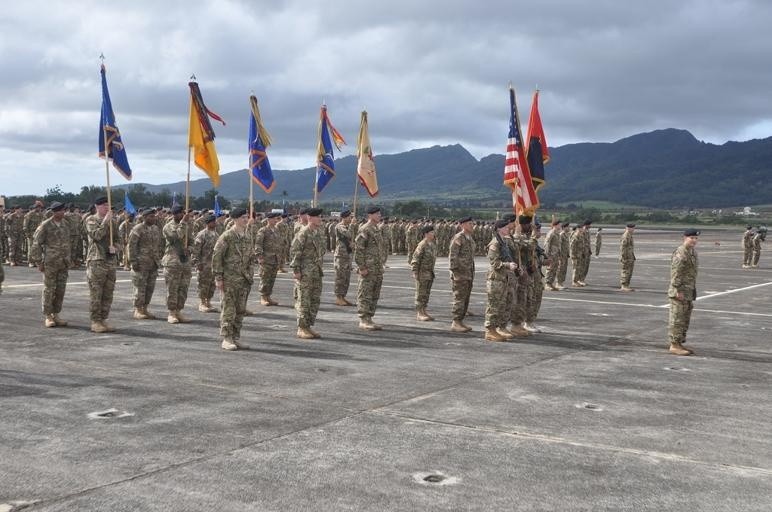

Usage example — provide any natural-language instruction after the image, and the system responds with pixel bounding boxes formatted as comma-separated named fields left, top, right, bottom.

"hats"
left=459, top=217, right=472, bottom=222
left=582, top=219, right=591, bottom=225
left=576, top=223, right=582, bottom=228
left=756, top=230, right=761, bottom=233
left=206, top=215, right=216, bottom=223
left=561, top=222, right=569, bottom=227
left=503, top=214, right=515, bottom=222
left=519, top=216, right=532, bottom=223
left=597, top=228, right=601, bottom=231
left=368, top=207, right=380, bottom=214
left=340, top=209, right=350, bottom=217
left=747, top=226, right=752, bottom=230
left=300, top=207, right=308, bottom=213
left=552, top=221, right=560, bottom=225
left=422, top=227, right=434, bottom=232
left=307, top=208, right=323, bottom=216
left=536, top=224, right=541, bottom=229
left=495, top=220, right=508, bottom=230
left=265, top=212, right=277, bottom=218
left=94, top=195, right=108, bottom=204
left=229, top=208, right=246, bottom=218
left=281, top=215, right=287, bottom=218
left=626, top=223, right=635, bottom=228
left=683, top=229, right=701, bottom=236
left=171, top=204, right=183, bottom=212
left=142, top=209, right=154, bottom=215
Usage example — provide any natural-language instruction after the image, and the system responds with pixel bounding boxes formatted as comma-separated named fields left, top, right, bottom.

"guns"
left=495, top=234, right=520, bottom=279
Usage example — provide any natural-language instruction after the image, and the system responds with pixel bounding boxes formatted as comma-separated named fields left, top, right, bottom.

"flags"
left=520, top=91, right=550, bottom=196
left=502, top=87, right=541, bottom=218
left=313, top=105, right=346, bottom=193
left=246, top=94, right=276, bottom=193
left=356, top=109, right=378, bottom=197
left=97, top=63, right=133, bottom=178
left=187, top=82, right=227, bottom=187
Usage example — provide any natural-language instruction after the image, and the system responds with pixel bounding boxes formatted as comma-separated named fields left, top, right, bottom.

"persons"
left=752, top=230, right=762, bottom=267
left=619, top=223, right=636, bottom=292
left=0, top=198, right=496, bottom=276
left=446, top=217, right=477, bottom=331
left=353, top=206, right=386, bottom=331
left=31, top=196, right=221, bottom=335
left=211, top=207, right=356, bottom=352
left=410, top=226, right=438, bottom=321
left=594, top=228, right=602, bottom=259
left=742, top=225, right=755, bottom=268
left=483, top=213, right=593, bottom=341
left=666, top=227, right=701, bottom=357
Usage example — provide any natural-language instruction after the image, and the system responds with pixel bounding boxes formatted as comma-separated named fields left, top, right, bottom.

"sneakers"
left=555, top=283, right=567, bottom=290
left=233, top=340, right=249, bottom=349
left=207, top=303, right=217, bottom=311
left=669, top=342, right=690, bottom=355
left=45, top=314, right=56, bottom=327
left=335, top=295, right=347, bottom=305
left=620, top=285, right=634, bottom=291
left=133, top=306, right=147, bottom=318
left=51, top=202, right=65, bottom=211
left=544, top=284, right=554, bottom=291
left=143, top=308, right=154, bottom=318
left=461, top=323, right=472, bottom=330
left=511, top=326, right=528, bottom=337
left=261, top=295, right=270, bottom=305
left=222, top=336, right=236, bottom=350
left=168, top=311, right=180, bottom=323
left=450, top=320, right=467, bottom=332
left=524, top=326, right=539, bottom=333
left=53, top=315, right=67, bottom=326
left=199, top=303, right=211, bottom=312
left=417, top=312, right=429, bottom=321
left=359, top=319, right=376, bottom=330
left=268, top=296, right=278, bottom=305
left=177, top=312, right=192, bottom=322
left=496, top=329, right=514, bottom=339
left=297, top=327, right=314, bottom=339
left=485, top=332, right=504, bottom=341
left=90, top=321, right=105, bottom=333
left=578, top=281, right=586, bottom=286
left=681, top=344, right=693, bottom=353
left=103, top=321, right=115, bottom=331
left=369, top=319, right=383, bottom=329
left=572, top=282, right=582, bottom=286
left=307, top=328, right=320, bottom=338
